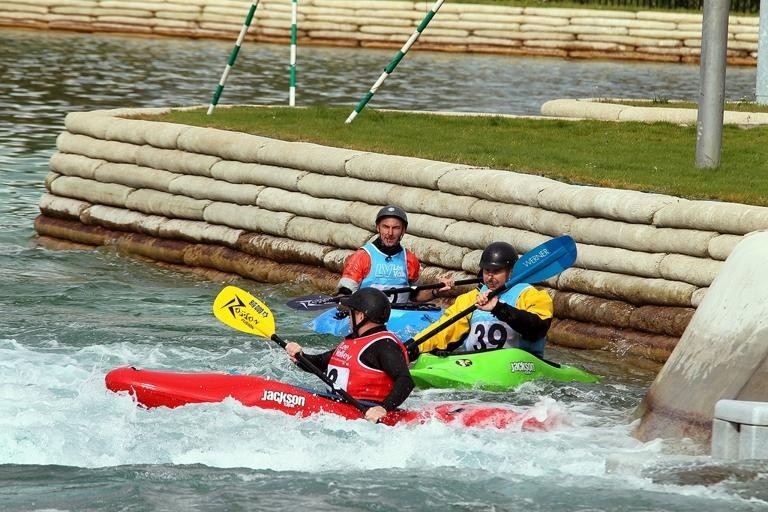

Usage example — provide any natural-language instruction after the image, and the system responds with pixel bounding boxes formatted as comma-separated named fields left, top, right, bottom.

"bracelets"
left=436, top=289, right=440, bottom=295
left=430, top=288, right=435, bottom=297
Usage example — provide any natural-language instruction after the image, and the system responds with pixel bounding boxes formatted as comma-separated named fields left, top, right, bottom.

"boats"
left=302, top=307, right=447, bottom=344
left=409, top=347, right=604, bottom=391
left=106, top=367, right=566, bottom=431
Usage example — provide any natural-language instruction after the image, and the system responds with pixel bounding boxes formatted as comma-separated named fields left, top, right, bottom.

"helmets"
left=343, top=287, right=391, bottom=323
left=479, top=241, right=519, bottom=271
left=375, top=204, right=407, bottom=225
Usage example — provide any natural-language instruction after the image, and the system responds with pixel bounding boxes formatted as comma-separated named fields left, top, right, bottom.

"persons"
left=403, top=241, right=554, bottom=363
left=334, top=205, right=456, bottom=307
left=284, top=288, right=415, bottom=424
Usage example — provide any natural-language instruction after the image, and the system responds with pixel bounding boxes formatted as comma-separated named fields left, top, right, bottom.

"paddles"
left=288, top=277, right=483, bottom=312
left=212, top=284, right=383, bottom=426
left=405, top=235, right=578, bottom=349
